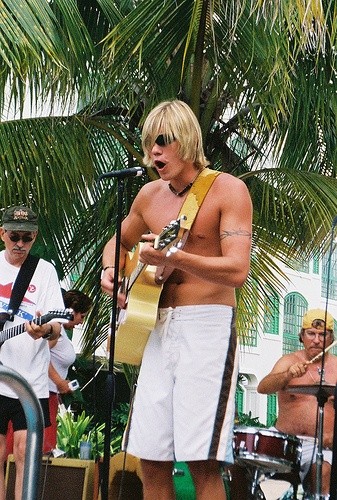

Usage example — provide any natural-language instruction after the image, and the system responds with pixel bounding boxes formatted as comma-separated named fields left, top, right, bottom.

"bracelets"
left=104, top=266, right=115, bottom=271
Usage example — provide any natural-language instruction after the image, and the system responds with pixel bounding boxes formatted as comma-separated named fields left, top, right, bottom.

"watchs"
left=42, top=325, right=55, bottom=340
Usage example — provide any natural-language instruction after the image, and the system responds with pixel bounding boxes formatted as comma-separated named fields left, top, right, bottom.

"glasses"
left=144, top=133, right=175, bottom=149
left=9, top=235, right=36, bottom=243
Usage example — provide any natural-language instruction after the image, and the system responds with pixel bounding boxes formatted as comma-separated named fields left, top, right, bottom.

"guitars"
left=0, top=308, right=75, bottom=348
left=105, top=216, right=188, bottom=366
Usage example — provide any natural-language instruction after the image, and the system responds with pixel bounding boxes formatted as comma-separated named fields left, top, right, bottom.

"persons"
left=100, top=101, right=252, bottom=500
left=0, top=206, right=68, bottom=500
left=256, top=309, right=337, bottom=500
left=5, top=288, right=92, bottom=462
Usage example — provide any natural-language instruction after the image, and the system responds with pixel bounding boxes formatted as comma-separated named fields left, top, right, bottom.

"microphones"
left=98, top=166, right=148, bottom=178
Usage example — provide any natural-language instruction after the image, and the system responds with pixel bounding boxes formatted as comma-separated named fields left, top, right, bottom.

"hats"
left=1, top=206, right=38, bottom=232
left=302, top=308, right=335, bottom=332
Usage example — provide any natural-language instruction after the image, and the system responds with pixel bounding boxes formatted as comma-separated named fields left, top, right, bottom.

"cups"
left=80, top=442, right=91, bottom=459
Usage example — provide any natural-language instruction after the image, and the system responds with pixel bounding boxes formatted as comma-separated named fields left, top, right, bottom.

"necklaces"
left=168, top=166, right=205, bottom=196
left=318, top=369, right=321, bottom=375
left=308, top=370, right=319, bottom=382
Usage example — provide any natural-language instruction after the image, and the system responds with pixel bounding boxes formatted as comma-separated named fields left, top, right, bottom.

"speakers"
left=5, top=454, right=97, bottom=500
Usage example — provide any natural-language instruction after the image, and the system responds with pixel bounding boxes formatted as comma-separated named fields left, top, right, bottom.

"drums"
left=233, top=427, right=297, bottom=474
left=220, top=464, right=265, bottom=500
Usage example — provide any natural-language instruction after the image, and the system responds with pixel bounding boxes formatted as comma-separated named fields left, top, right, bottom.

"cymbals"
left=284, top=383, right=336, bottom=397
